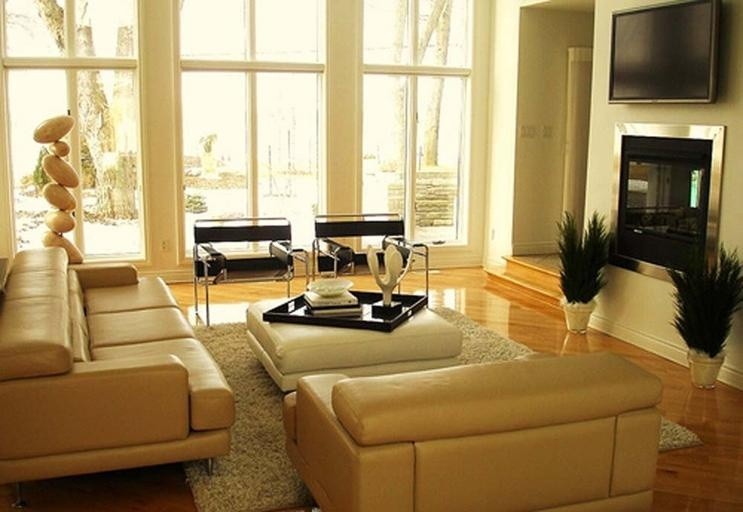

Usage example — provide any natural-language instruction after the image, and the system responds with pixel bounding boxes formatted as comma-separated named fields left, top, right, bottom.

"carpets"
left=182, top=307, right=704, bottom=510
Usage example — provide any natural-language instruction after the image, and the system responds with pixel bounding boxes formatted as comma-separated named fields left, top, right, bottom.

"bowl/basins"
left=307, top=278, right=354, bottom=297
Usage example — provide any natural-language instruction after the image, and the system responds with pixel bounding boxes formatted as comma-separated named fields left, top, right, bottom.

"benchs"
left=191, top=214, right=296, bottom=285
left=310, top=212, right=414, bottom=281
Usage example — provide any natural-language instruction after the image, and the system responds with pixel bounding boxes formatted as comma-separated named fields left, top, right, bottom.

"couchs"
left=279, top=345, right=668, bottom=510
left=0, top=248, right=236, bottom=506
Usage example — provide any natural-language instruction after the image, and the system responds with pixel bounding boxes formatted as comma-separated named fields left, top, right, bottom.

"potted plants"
left=549, top=206, right=616, bottom=335
left=665, top=240, right=743, bottom=390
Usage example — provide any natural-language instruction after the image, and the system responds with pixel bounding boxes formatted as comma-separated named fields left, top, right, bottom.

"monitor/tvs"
left=607, top=0, right=725, bottom=108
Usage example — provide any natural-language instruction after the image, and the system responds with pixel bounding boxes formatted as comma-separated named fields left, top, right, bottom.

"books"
left=304, top=290, right=362, bottom=317
